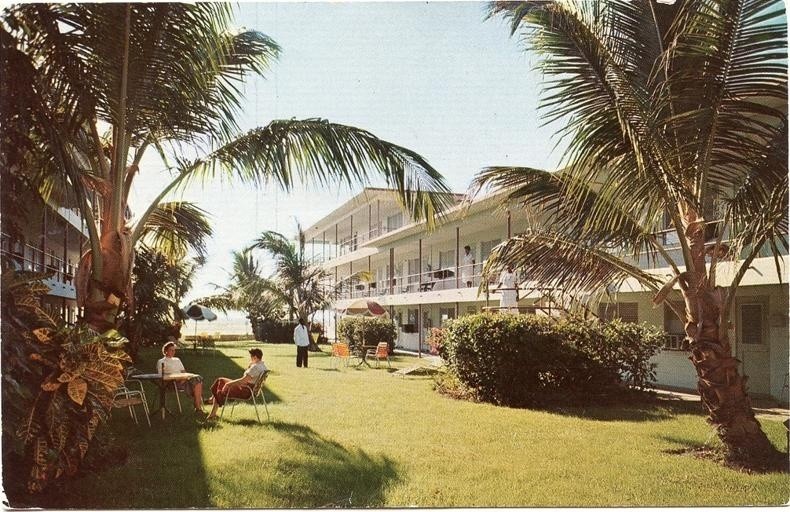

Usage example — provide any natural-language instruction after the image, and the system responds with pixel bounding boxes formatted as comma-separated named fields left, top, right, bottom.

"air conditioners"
left=663, top=335, right=684, bottom=351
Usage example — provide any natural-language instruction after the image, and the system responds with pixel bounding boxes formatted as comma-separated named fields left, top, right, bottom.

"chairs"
left=150, top=378, right=197, bottom=416
left=103, top=366, right=151, bottom=428
left=355, top=276, right=435, bottom=294
left=166, top=334, right=215, bottom=360
left=218, top=370, right=271, bottom=426
left=365, top=342, right=391, bottom=369
left=329, top=342, right=359, bottom=371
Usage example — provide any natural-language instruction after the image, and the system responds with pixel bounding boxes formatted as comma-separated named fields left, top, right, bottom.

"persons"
left=203, top=348, right=267, bottom=422
left=292, top=317, right=311, bottom=368
left=156, top=341, right=209, bottom=416
left=461, top=245, right=476, bottom=287
left=491, top=263, right=520, bottom=316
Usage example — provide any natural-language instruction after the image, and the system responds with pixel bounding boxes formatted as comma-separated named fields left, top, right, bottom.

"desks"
left=349, top=345, right=376, bottom=369
left=130, top=373, right=199, bottom=420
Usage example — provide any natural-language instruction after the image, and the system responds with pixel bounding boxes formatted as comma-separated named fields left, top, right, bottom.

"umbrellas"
left=182, top=301, right=217, bottom=342
left=339, top=296, right=391, bottom=345
